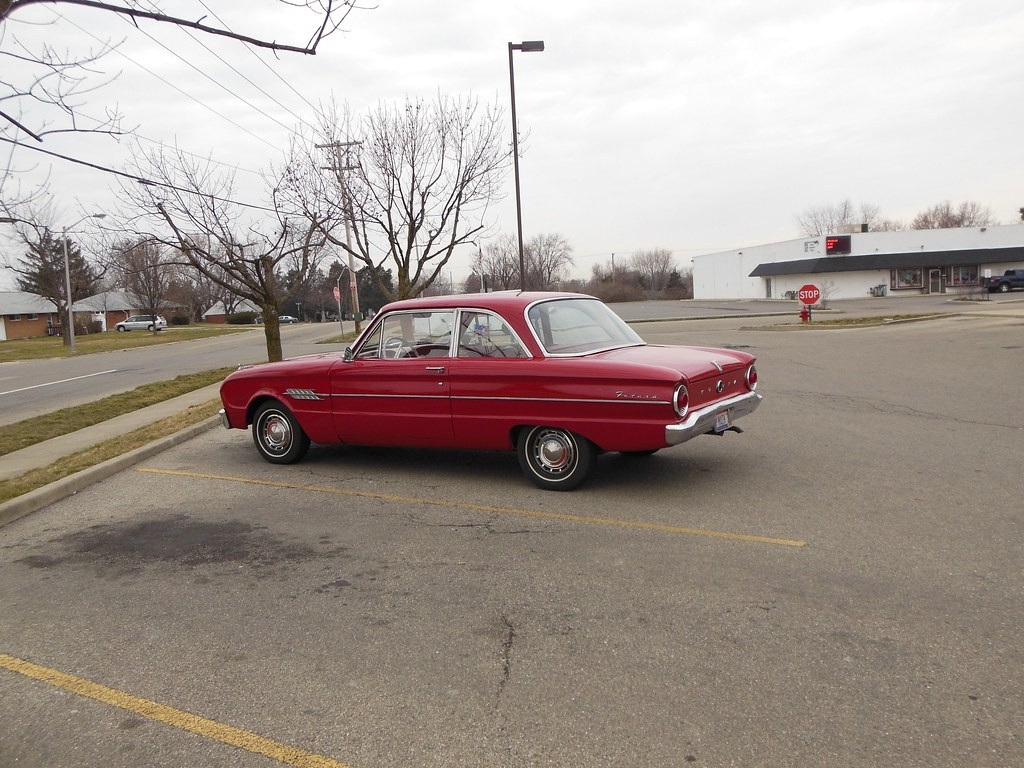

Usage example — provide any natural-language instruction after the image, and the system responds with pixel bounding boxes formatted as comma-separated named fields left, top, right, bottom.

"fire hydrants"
left=799, top=306, right=810, bottom=323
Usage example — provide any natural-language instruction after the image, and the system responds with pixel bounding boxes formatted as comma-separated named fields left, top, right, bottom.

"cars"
left=255, top=317, right=264, bottom=324
left=784, top=290, right=799, bottom=301
left=217, top=288, right=766, bottom=493
left=278, top=316, right=299, bottom=324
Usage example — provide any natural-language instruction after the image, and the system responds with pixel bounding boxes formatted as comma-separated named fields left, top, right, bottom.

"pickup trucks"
left=982, top=269, right=1024, bottom=294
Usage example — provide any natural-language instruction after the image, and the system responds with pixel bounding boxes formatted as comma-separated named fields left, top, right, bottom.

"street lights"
left=508, top=41, right=545, bottom=291
left=62, top=213, right=107, bottom=352
left=337, top=266, right=350, bottom=338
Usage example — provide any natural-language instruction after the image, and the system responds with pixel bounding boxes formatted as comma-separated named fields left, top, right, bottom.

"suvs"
left=114, top=314, right=168, bottom=333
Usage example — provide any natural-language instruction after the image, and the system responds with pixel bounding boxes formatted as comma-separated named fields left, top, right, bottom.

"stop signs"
left=799, top=284, right=822, bottom=305
